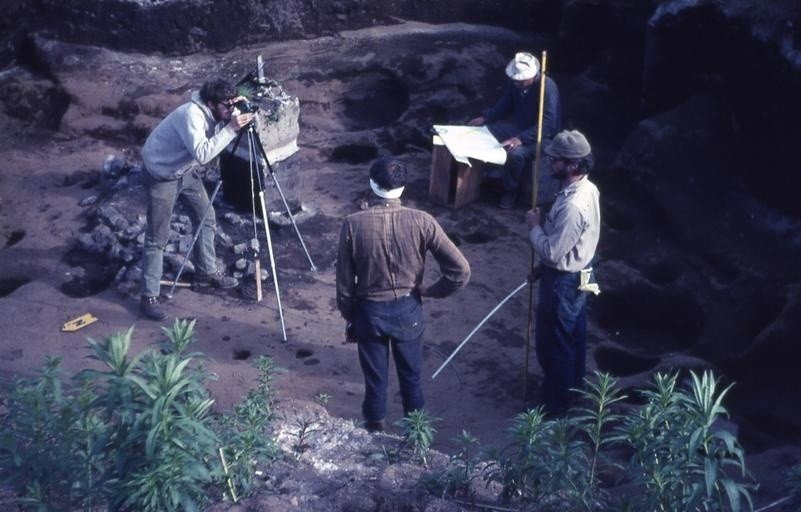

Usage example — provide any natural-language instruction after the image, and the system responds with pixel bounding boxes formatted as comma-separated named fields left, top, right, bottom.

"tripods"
left=166, top=127, right=318, bottom=342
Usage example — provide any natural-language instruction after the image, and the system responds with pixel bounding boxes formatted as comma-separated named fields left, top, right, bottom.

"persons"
left=335, top=157, right=472, bottom=432
left=139, top=77, right=255, bottom=322
left=525, top=130, right=602, bottom=419
left=467, top=51, right=562, bottom=209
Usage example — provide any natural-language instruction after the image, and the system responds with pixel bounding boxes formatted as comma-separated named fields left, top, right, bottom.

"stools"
left=518, top=152, right=563, bottom=205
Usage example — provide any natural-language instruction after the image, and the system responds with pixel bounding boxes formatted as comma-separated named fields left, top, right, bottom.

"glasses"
left=545, top=155, right=563, bottom=161
left=218, top=101, right=234, bottom=109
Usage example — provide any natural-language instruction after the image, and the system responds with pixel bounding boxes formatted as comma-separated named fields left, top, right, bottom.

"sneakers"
left=141, top=295, right=168, bottom=321
left=194, top=271, right=239, bottom=289
left=498, top=190, right=518, bottom=208
left=520, top=399, right=569, bottom=419
left=357, top=419, right=389, bottom=434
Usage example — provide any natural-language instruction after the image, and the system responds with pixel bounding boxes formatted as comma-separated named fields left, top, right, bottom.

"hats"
left=545, top=129, right=592, bottom=159
left=505, top=51, right=541, bottom=82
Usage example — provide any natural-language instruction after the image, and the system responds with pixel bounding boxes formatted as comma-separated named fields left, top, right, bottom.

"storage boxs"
left=428, top=138, right=482, bottom=209
